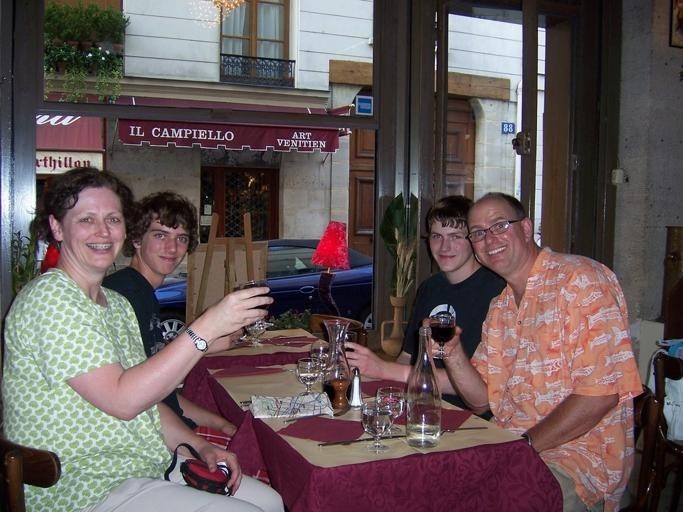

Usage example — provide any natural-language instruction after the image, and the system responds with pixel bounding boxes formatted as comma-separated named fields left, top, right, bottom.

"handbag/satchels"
left=180, top=459, right=233, bottom=496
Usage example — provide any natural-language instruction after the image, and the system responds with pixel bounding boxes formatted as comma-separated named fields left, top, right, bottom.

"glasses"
left=465, top=220, right=520, bottom=243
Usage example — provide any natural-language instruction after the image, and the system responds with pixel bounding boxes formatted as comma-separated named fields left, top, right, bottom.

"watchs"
left=185, top=328, right=208, bottom=353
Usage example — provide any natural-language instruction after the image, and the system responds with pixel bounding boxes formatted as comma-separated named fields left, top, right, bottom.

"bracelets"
left=520, top=433, right=532, bottom=446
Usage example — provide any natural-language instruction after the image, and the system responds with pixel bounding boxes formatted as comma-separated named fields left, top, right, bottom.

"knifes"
left=317, top=435, right=404, bottom=447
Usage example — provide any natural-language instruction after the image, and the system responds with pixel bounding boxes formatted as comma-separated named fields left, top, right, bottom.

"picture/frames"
left=669, top=0, right=683, bottom=49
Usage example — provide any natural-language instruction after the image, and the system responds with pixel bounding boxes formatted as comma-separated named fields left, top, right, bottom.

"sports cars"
left=142, top=239, right=377, bottom=337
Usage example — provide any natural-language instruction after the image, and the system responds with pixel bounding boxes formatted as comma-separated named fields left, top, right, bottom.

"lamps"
left=310, top=220, right=351, bottom=316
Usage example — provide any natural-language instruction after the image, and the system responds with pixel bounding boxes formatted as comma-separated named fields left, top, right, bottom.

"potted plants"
left=379, top=191, right=418, bottom=357
left=44, top=0, right=131, bottom=105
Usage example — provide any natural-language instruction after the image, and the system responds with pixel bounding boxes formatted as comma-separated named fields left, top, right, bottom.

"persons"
left=100, top=190, right=237, bottom=437
left=422, top=191, right=646, bottom=511
left=2, top=166, right=285, bottom=512
left=344, top=194, right=508, bottom=421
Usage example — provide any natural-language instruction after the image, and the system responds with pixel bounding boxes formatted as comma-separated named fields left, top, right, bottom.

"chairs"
left=309, top=313, right=365, bottom=345
left=0, top=424, right=61, bottom=512
left=616, top=383, right=660, bottom=512
left=647, top=350, right=683, bottom=512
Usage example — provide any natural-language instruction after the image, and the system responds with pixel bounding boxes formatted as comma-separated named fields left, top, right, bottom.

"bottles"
left=406, top=327, right=442, bottom=450
left=323, top=319, right=353, bottom=401
left=329, top=367, right=364, bottom=410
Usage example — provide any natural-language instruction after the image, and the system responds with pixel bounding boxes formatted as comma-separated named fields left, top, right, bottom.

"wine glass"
left=240, top=280, right=272, bottom=348
left=361, top=401, right=395, bottom=452
left=377, top=387, right=406, bottom=436
left=295, top=358, right=321, bottom=394
left=429, top=314, right=456, bottom=358
left=311, top=341, right=330, bottom=382
